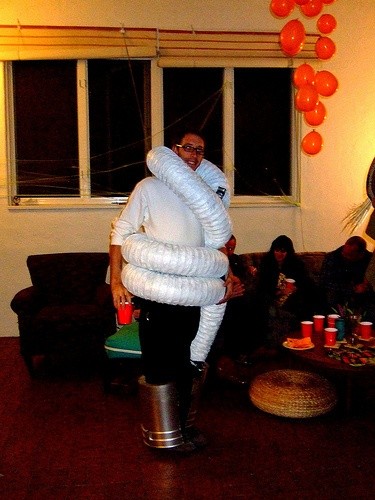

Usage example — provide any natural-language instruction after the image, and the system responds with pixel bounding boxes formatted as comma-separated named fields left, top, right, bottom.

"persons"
left=109, top=129, right=234, bottom=448
left=317, top=235, right=375, bottom=330
left=222, top=234, right=254, bottom=367
left=250, top=235, right=321, bottom=360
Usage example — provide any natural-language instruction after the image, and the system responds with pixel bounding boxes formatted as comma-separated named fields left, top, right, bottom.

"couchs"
left=217, top=251, right=375, bottom=384
left=10, top=251, right=118, bottom=383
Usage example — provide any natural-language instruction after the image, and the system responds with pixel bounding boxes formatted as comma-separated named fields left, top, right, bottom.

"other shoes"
left=177, top=424, right=211, bottom=448
left=166, top=440, right=198, bottom=454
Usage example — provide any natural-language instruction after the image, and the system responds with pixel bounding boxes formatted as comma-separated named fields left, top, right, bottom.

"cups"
left=300, top=321, right=314, bottom=338
left=313, top=315, right=325, bottom=332
left=324, top=328, right=338, bottom=346
left=117, top=301, right=134, bottom=325
left=360, top=321, right=373, bottom=340
left=284, top=278, right=295, bottom=296
left=328, top=315, right=340, bottom=328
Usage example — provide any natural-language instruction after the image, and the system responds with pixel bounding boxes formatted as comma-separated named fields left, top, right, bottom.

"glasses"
left=175, top=143, right=206, bottom=156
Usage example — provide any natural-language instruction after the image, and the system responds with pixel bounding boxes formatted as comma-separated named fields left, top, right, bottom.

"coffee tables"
left=278, top=330, right=375, bottom=414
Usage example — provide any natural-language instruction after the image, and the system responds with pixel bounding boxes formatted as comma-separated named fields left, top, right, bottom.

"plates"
left=282, top=341, right=315, bottom=350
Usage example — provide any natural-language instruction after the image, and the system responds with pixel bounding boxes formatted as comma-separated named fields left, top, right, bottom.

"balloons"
left=293, top=62, right=313, bottom=89
left=318, top=13, right=337, bottom=34
left=304, top=101, right=327, bottom=125
left=295, top=83, right=317, bottom=111
left=314, top=70, right=340, bottom=98
left=315, top=36, right=335, bottom=59
left=302, top=129, right=322, bottom=155
left=278, top=18, right=306, bottom=56
left=271, top=0, right=334, bottom=19
